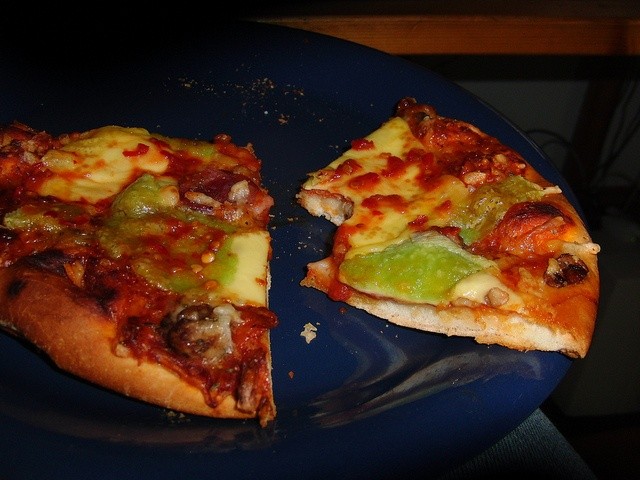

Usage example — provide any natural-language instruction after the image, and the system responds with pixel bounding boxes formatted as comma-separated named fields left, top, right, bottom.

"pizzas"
left=0, top=121, right=277, bottom=427
left=296, top=98, right=601, bottom=360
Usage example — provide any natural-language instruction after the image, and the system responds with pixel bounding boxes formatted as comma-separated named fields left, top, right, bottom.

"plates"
left=0, top=21, right=590, bottom=479
